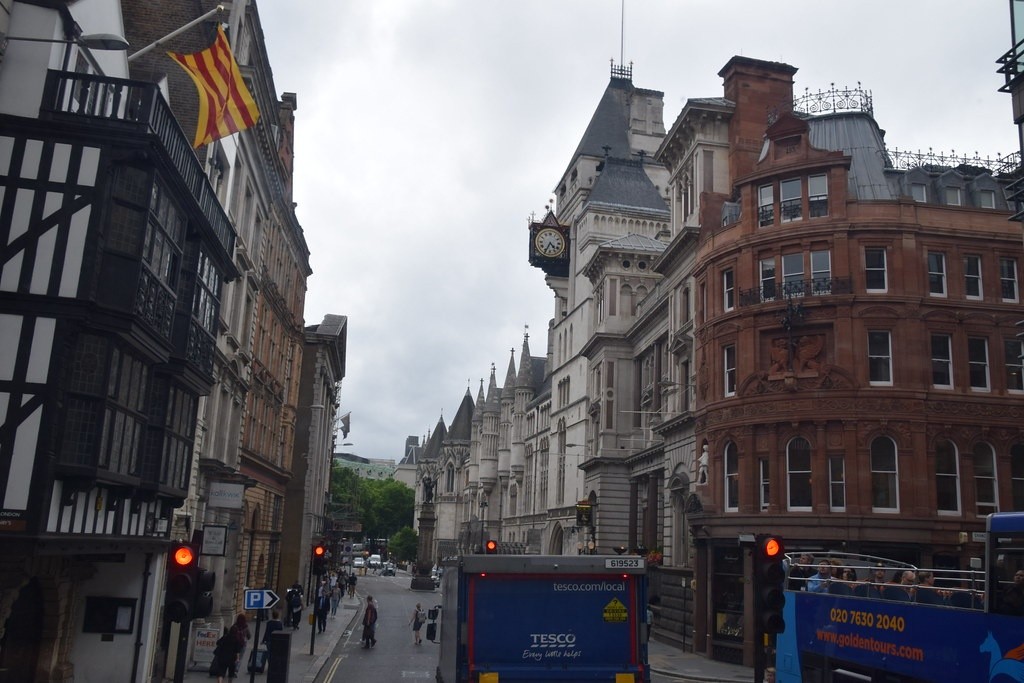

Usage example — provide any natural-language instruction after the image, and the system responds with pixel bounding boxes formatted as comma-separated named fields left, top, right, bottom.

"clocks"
left=536, top=228, right=565, bottom=258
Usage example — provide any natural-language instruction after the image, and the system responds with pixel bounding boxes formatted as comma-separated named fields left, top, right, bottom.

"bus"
left=774, top=512, right=1024, bottom=683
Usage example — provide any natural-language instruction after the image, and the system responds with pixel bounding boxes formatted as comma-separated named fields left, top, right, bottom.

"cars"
left=382, top=562, right=396, bottom=577
left=430, top=569, right=440, bottom=588
left=353, top=558, right=366, bottom=568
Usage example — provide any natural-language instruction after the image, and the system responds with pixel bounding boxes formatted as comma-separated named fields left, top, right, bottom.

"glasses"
left=906, top=578, right=915, bottom=583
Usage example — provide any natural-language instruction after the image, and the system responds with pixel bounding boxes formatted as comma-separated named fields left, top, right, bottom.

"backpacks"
left=416, top=609, right=425, bottom=623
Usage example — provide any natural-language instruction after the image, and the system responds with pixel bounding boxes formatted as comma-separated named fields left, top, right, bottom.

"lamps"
left=658, top=381, right=695, bottom=393
left=565, top=443, right=590, bottom=449
left=154, top=517, right=168, bottom=532
left=4, top=33, right=129, bottom=50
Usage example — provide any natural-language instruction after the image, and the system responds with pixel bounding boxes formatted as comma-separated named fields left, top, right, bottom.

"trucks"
left=426, top=553, right=650, bottom=683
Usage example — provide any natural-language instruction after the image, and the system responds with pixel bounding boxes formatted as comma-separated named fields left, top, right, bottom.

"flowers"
left=647, top=547, right=664, bottom=565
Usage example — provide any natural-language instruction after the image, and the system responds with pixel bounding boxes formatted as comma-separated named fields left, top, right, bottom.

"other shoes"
left=371, top=639, right=376, bottom=647
left=361, top=645, right=370, bottom=648
left=234, top=671, right=238, bottom=678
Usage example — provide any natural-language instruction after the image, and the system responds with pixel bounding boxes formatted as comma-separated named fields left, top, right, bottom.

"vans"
left=369, top=554, right=381, bottom=569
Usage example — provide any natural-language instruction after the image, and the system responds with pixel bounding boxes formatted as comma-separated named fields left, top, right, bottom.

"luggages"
left=247, top=643, right=267, bottom=673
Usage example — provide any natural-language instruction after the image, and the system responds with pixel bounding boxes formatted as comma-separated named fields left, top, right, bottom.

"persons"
left=788, top=553, right=1024, bottom=609
left=360, top=595, right=377, bottom=649
left=422, top=477, right=439, bottom=504
left=647, top=605, right=655, bottom=641
left=233, top=613, right=251, bottom=678
left=260, top=611, right=283, bottom=664
left=408, top=602, right=427, bottom=645
left=693, top=445, right=709, bottom=486
left=207, top=625, right=241, bottom=683
left=284, top=568, right=357, bottom=634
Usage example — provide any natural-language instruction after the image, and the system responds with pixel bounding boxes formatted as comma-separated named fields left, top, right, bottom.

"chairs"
left=827, top=580, right=982, bottom=608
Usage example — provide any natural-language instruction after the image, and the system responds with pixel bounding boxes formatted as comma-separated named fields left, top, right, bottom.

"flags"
left=340, top=426, right=347, bottom=439
left=164, top=22, right=261, bottom=151
left=340, top=413, right=350, bottom=432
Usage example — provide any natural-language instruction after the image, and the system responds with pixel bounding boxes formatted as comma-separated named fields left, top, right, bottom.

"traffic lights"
left=313, top=545, right=325, bottom=574
left=167, top=541, right=199, bottom=622
left=196, top=566, right=216, bottom=618
left=485, top=539, right=498, bottom=554
left=758, top=533, right=786, bottom=635
